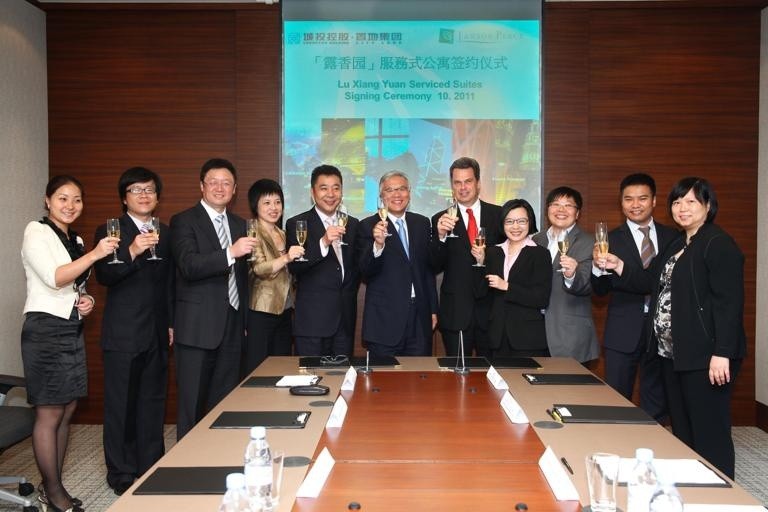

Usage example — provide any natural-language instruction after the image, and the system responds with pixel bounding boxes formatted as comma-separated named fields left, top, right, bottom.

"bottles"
left=220, top=473, right=248, bottom=512
left=649, top=473, right=682, bottom=512
left=625, top=445, right=659, bottom=512
left=244, top=424, right=273, bottom=511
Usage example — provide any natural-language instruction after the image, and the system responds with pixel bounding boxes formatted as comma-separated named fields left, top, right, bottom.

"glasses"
left=125, top=187, right=155, bottom=195
left=550, top=201, right=578, bottom=209
left=320, top=355, right=349, bottom=366
left=504, top=218, right=529, bottom=225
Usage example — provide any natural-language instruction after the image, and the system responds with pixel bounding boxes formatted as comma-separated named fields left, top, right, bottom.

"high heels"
left=37, top=481, right=85, bottom=512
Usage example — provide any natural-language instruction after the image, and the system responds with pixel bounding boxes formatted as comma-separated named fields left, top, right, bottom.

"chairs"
left=2, top=370, right=54, bottom=511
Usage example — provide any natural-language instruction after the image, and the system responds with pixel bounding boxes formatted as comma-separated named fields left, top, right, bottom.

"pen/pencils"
left=562, top=458, right=574, bottom=475
left=460, top=330, right=466, bottom=371
left=546, top=408, right=562, bottom=421
left=366, top=350, right=370, bottom=372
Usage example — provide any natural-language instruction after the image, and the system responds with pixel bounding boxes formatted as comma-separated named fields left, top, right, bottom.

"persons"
left=169, top=158, right=257, bottom=441
left=531, top=186, right=600, bottom=368
left=354, top=169, right=438, bottom=356
left=95, top=167, right=174, bottom=496
left=592, top=174, right=680, bottom=427
left=245, top=179, right=305, bottom=375
left=286, top=164, right=360, bottom=357
left=21, top=176, right=120, bottom=511
left=430, top=157, right=507, bottom=358
left=470, top=199, right=553, bottom=357
left=598, top=177, right=748, bottom=481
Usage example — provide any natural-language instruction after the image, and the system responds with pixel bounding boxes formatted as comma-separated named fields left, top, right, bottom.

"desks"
left=103, top=352, right=767, bottom=511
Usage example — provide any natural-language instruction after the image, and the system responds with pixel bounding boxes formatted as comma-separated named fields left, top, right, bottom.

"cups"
left=585, top=450, right=620, bottom=511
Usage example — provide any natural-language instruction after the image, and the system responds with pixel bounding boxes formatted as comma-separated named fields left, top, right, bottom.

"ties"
left=639, top=226, right=656, bottom=308
left=216, top=215, right=239, bottom=311
left=326, top=218, right=344, bottom=280
left=466, top=208, right=479, bottom=251
left=395, top=218, right=410, bottom=264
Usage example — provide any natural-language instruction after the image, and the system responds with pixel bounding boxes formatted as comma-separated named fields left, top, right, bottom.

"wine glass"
left=471, top=227, right=487, bottom=268
left=592, top=222, right=613, bottom=276
left=245, top=218, right=261, bottom=262
left=444, top=197, right=461, bottom=238
left=555, top=228, right=573, bottom=274
left=376, top=197, right=393, bottom=237
left=106, top=217, right=124, bottom=266
left=294, top=219, right=309, bottom=262
left=335, top=206, right=348, bottom=246
left=146, top=215, right=164, bottom=261
